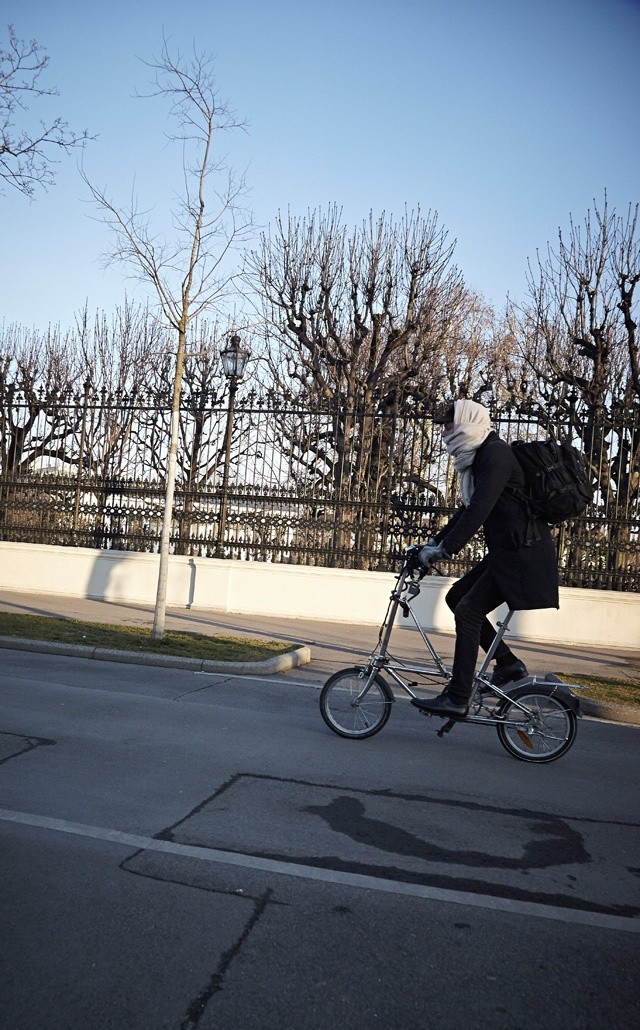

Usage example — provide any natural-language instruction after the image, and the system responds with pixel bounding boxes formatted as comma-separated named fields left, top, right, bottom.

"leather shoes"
left=410, top=695, right=470, bottom=721
left=478, top=659, right=529, bottom=693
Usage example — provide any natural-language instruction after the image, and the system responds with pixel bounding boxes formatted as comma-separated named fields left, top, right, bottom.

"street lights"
left=211, top=332, right=252, bottom=563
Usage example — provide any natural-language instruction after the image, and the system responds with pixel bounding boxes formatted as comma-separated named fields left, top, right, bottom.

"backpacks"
left=477, top=437, right=594, bottom=525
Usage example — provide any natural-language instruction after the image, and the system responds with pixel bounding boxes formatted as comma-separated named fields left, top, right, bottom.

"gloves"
left=406, top=538, right=444, bottom=568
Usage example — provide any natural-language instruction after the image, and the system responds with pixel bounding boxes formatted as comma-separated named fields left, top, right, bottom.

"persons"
left=409, top=401, right=559, bottom=720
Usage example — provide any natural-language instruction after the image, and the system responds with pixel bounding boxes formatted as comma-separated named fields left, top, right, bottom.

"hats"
left=431, top=403, right=454, bottom=424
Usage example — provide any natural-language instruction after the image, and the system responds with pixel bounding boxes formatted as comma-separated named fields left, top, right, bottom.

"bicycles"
left=318, top=544, right=586, bottom=765
